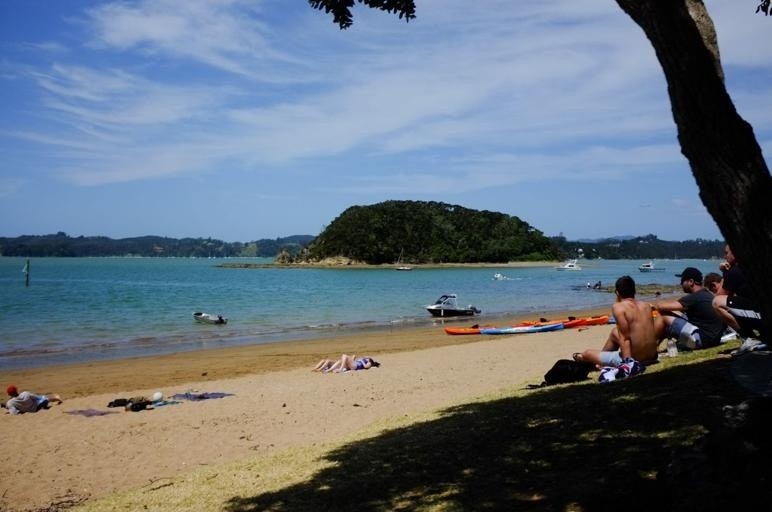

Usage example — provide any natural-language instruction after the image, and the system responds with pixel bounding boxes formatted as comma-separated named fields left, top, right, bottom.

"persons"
left=321, top=354, right=380, bottom=373
left=571, top=238, right=762, bottom=368
left=311, top=354, right=356, bottom=372
left=7, top=384, right=64, bottom=414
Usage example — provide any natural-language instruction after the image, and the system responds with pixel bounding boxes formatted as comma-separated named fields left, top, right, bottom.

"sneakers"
left=731, top=338, right=765, bottom=356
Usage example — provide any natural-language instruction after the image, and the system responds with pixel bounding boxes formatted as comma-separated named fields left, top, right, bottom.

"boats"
left=395, top=266, right=412, bottom=270
left=638, top=263, right=654, bottom=270
left=493, top=273, right=507, bottom=280
left=192, top=311, right=228, bottom=325
left=425, top=293, right=474, bottom=316
left=442, top=315, right=609, bottom=335
left=556, top=262, right=582, bottom=271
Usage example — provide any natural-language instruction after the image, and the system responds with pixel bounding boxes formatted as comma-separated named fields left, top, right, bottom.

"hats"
left=675, top=267, right=702, bottom=279
left=7, top=385, right=17, bottom=395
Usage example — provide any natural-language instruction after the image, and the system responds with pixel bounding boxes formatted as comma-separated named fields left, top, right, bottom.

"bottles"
left=667, top=336, right=679, bottom=360
